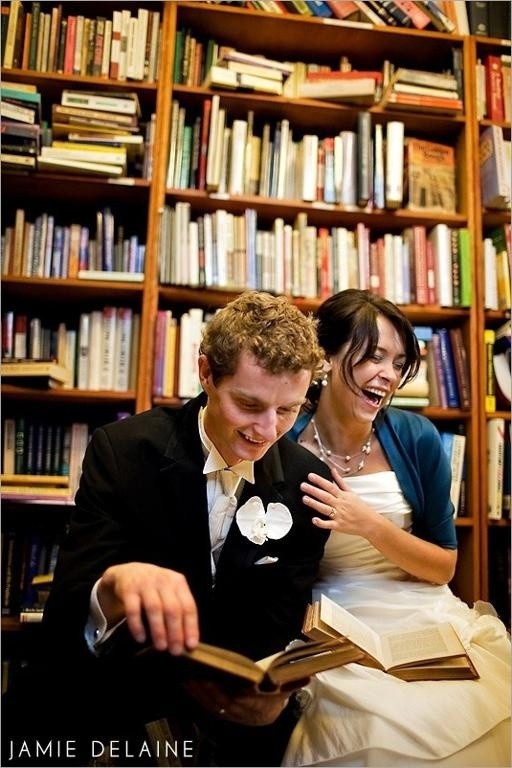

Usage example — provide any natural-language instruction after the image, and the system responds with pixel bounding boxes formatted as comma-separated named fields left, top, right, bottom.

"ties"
left=209, top=471, right=241, bottom=577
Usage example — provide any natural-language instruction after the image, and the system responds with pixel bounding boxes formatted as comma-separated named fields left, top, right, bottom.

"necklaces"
left=312, top=418, right=376, bottom=478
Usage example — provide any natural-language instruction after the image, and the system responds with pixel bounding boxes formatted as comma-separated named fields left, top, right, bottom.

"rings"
left=329, top=507, right=337, bottom=519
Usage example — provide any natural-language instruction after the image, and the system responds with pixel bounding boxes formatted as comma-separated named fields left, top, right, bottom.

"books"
left=302, top=594, right=480, bottom=682
left=171, top=28, right=464, bottom=114
left=474, top=53, right=512, bottom=123
left=479, top=126, right=512, bottom=212
left=386, top=324, right=470, bottom=411
left=1, top=1, right=165, bottom=83
left=149, top=204, right=472, bottom=308
left=1, top=536, right=60, bottom=623
left=2, top=659, right=27, bottom=694
left=151, top=304, right=224, bottom=400
left=485, top=418, right=512, bottom=521
left=481, top=223, right=512, bottom=313
left=1, top=412, right=131, bottom=500
left=163, top=93, right=458, bottom=215
left=0, top=82, right=157, bottom=181
left=136, top=634, right=367, bottom=698
left=1, top=305, right=141, bottom=393
left=175, top=1, right=457, bottom=40
left=483, top=321, right=511, bottom=413
left=0, top=207, right=147, bottom=283
left=438, top=432, right=469, bottom=518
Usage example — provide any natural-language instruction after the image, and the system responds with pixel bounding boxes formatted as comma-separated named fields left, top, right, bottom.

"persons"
left=279, top=288, right=512, bottom=768
left=1, top=290, right=335, bottom=767
